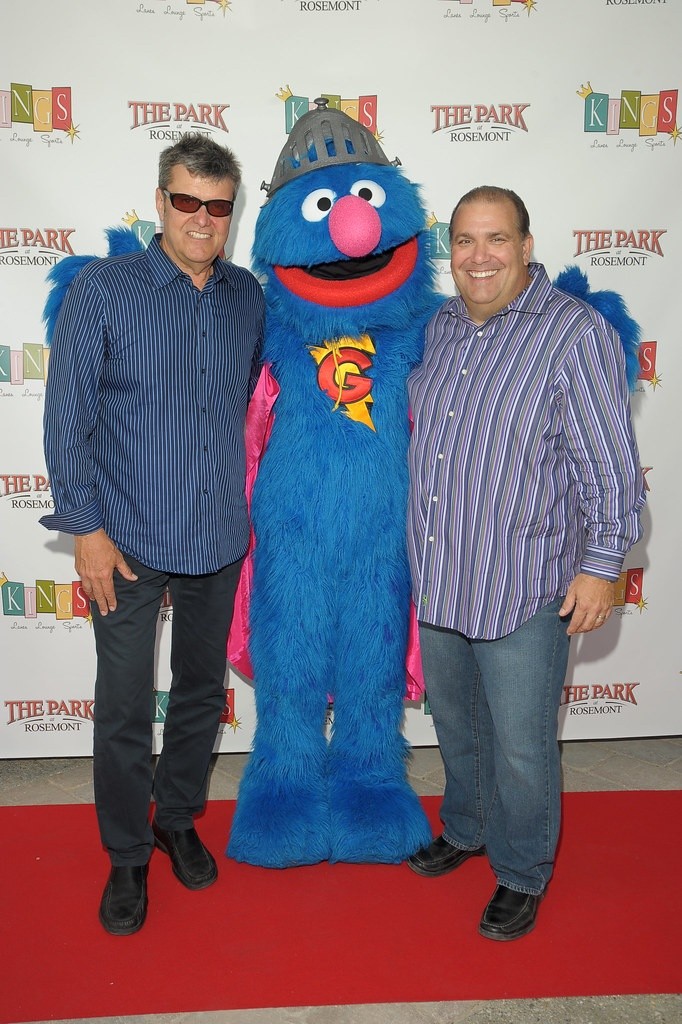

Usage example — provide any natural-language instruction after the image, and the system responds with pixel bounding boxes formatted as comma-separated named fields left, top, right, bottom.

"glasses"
left=164, top=190, right=233, bottom=217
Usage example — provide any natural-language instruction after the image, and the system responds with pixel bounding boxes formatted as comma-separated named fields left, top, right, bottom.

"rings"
left=597, top=615, right=606, bottom=619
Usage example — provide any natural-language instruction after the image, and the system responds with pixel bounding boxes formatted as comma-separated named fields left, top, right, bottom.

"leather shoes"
left=408, top=836, right=485, bottom=877
left=98, top=865, right=148, bottom=937
left=479, top=883, right=542, bottom=941
left=151, top=814, right=217, bottom=890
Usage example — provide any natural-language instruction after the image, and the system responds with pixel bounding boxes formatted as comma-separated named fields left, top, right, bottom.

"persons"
left=408, top=185, right=648, bottom=941
left=39, top=134, right=267, bottom=935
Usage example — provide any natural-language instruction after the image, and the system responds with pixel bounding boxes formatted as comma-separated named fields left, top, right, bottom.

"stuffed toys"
left=43, top=97, right=642, bottom=870
